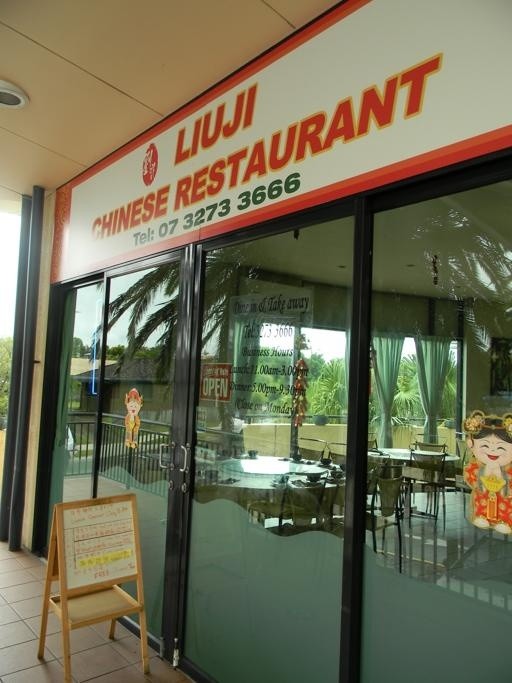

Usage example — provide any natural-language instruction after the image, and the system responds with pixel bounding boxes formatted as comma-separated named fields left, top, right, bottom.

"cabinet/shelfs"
left=36, top=494, right=150, bottom=678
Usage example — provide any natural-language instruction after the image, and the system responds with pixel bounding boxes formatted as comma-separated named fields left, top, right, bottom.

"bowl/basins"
left=293, top=455, right=342, bottom=483
left=248, top=450, right=258, bottom=457
left=205, top=470, right=218, bottom=481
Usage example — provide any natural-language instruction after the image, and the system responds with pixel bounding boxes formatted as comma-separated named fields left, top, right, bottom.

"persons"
left=124, top=387, right=143, bottom=448
left=461, top=408, right=512, bottom=534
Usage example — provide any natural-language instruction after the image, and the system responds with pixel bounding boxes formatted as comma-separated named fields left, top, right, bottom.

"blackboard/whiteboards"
left=55, top=492, right=143, bottom=597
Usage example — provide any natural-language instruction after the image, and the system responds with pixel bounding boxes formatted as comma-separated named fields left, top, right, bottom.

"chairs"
left=193, top=441, right=461, bottom=575
